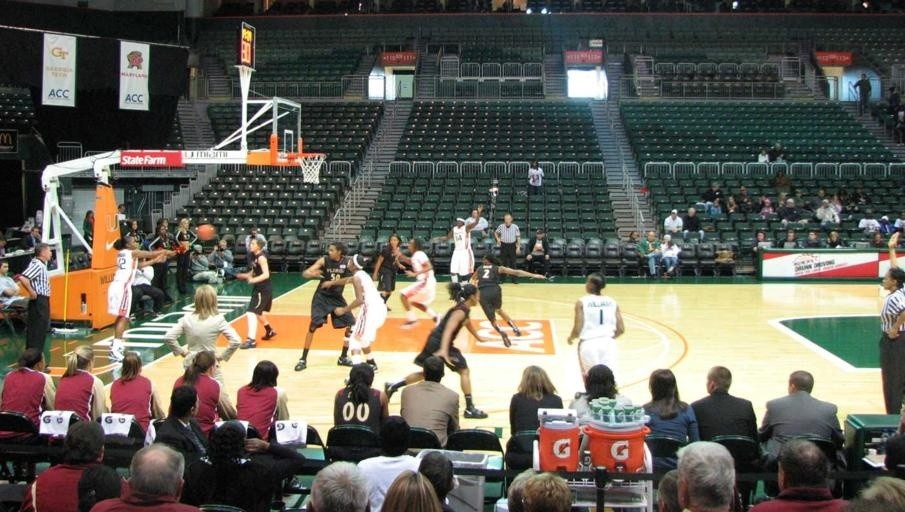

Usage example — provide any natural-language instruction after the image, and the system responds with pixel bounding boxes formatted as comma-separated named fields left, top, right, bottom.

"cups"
left=587, top=396, right=645, bottom=424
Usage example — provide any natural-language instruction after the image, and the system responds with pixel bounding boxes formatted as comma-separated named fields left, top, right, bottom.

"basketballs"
left=197, top=225, right=216, bottom=242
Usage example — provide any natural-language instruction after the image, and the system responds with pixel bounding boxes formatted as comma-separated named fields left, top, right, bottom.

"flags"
left=40, top=34, right=75, bottom=108
left=119, top=40, right=151, bottom=112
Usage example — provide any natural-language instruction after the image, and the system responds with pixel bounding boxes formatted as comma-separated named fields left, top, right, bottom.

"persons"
left=83, top=211, right=94, bottom=248
left=568, top=274, right=624, bottom=383
left=885, top=87, right=900, bottom=117
left=308, top=356, right=461, bottom=511
left=629, top=185, right=904, bottom=278
left=295, top=242, right=353, bottom=369
left=384, top=284, right=488, bottom=418
left=235, top=240, right=276, bottom=349
left=505, top=364, right=904, bottom=512
left=894, top=105, right=905, bottom=144
left=878, top=232, right=905, bottom=415
left=321, top=252, right=388, bottom=380
left=1, top=345, right=311, bottom=511
left=117, top=204, right=267, bottom=315
left=165, top=285, right=241, bottom=383
left=372, top=203, right=549, bottom=328
left=0, top=228, right=42, bottom=315
left=20, top=243, right=52, bottom=352
left=854, top=73, right=871, bottom=116
left=528, top=162, right=545, bottom=193
left=105, top=237, right=177, bottom=363
left=467, top=255, right=546, bottom=345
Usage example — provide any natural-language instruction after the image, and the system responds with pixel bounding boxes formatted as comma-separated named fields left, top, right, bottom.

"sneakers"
left=402, top=321, right=418, bottom=328
left=501, top=332, right=511, bottom=347
left=514, top=328, right=520, bottom=336
left=109, top=342, right=126, bottom=361
left=385, top=382, right=392, bottom=400
left=241, top=338, right=255, bottom=349
left=464, top=408, right=486, bottom=418
left=433, top=315, right=440, bottom=327
left=337, top=358, right=378, bottom=384
left=296, top=360, right=306, bottom=370
left=262, top=331, right=275, bottom=340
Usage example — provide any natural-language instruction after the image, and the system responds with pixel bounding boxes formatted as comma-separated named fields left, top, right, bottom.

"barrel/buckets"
left=588, top=416, right=650, bottom=431
left=539, top=424, right=580, bottom=471
left=585, top=428, right=651, bottom=480
